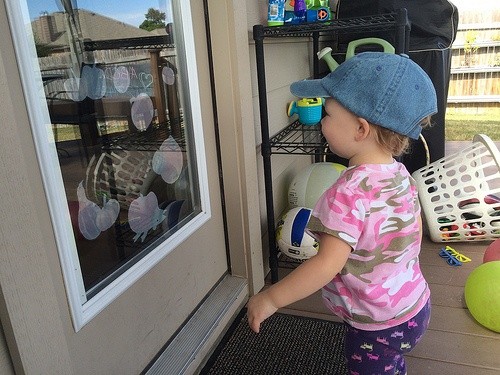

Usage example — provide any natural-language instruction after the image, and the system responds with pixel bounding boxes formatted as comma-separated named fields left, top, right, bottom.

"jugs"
left=317, top=38, right=395, bottom=73
left=287, top=97, right=322, bottom=125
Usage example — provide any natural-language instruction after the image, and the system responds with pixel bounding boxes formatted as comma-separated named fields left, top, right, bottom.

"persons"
left=245, top=51, right=437, bottom=375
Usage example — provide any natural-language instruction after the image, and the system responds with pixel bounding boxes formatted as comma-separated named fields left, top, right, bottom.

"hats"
left=290, top=51, right=438, bottom=141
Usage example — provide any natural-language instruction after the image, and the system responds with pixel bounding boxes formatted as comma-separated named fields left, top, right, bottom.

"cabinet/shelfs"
left=252, top=8, right=412, bottom=281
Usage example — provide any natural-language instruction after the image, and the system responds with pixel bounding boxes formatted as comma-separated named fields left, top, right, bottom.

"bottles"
left=284, top=10, right=307, bottom=24
left=267, top=0, right=285, bottom=26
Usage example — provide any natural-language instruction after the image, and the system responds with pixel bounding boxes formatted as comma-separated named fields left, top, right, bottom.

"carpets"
left=198, top=307, right=351, bottom=375
